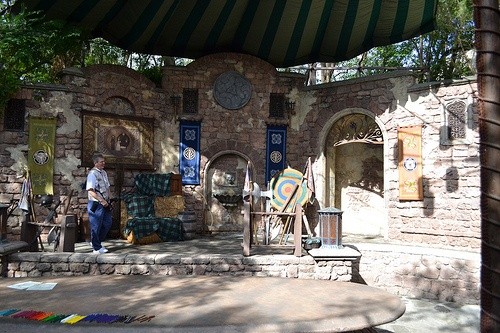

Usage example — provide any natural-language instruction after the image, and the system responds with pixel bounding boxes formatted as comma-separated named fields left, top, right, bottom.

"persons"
left=85, top=153, right=113, bottom=253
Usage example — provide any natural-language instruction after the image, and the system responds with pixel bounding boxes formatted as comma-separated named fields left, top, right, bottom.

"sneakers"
left=93, top=247, right=108, bottom=253
left=89, top=242, right=92, bottom=246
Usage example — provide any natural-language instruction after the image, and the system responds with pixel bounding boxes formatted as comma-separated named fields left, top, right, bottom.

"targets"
left=269, top=167, right=311, bottom=213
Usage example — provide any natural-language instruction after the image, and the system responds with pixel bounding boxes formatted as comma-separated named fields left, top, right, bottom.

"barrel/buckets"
left=177, top=211, right=196, bottom=240
